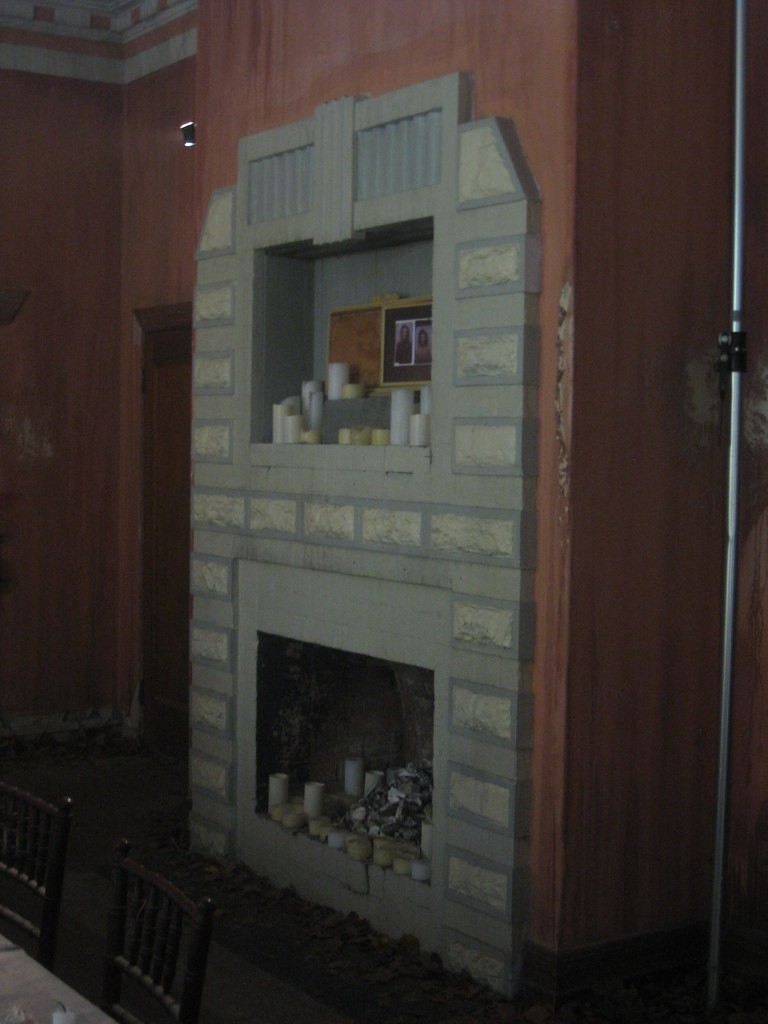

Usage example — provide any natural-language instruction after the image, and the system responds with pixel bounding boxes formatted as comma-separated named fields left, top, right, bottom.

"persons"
left=415, top=331, right=431, bottom=363
left=396, top=325, right=412, bottom=364
